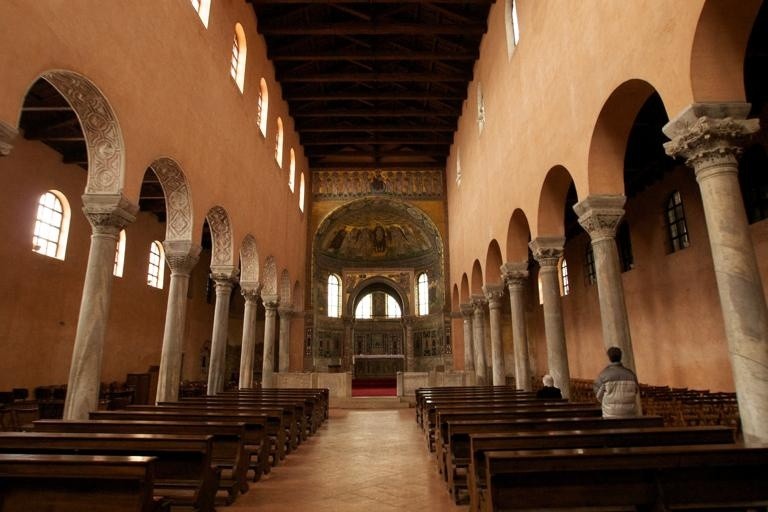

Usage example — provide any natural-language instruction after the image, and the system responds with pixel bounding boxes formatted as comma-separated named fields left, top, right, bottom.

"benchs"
left=416, top=385, right=767, bottom=512
left=1, top=385, right=330, bottom=511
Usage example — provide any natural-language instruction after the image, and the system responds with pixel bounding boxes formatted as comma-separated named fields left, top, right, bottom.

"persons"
left=591, top=347, right=640, bottom=419
left=535, top=375, right=561, bottom=399
